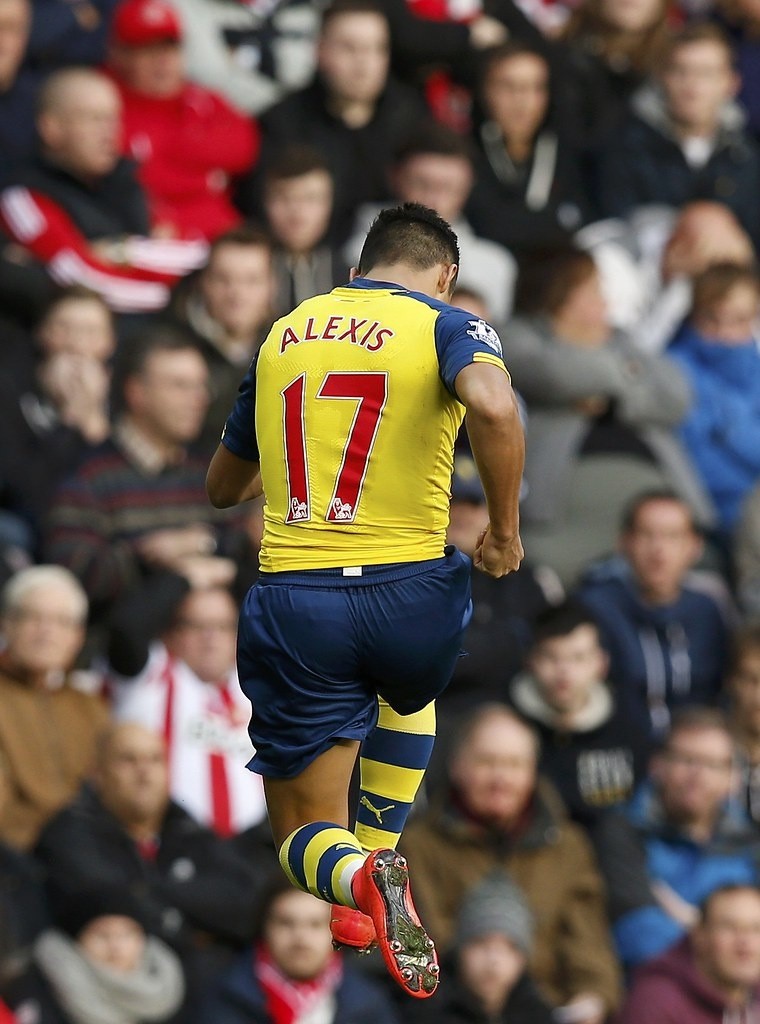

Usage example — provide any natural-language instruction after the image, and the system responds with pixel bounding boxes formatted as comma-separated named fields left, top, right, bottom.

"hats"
left=449, top=874, right=543, bottom=960
left=61, top=879, right=154, bottom=949
left=112, top=1, right=185, bottom=53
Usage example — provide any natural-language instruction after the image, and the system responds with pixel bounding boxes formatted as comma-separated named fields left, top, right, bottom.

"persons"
left=0, top=1, right=760, bottom=1024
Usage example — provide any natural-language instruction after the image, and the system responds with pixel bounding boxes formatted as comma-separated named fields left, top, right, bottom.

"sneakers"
left=326, top=901, right=377, bottom=956
left=349, top=848, right=441, bottom=999
